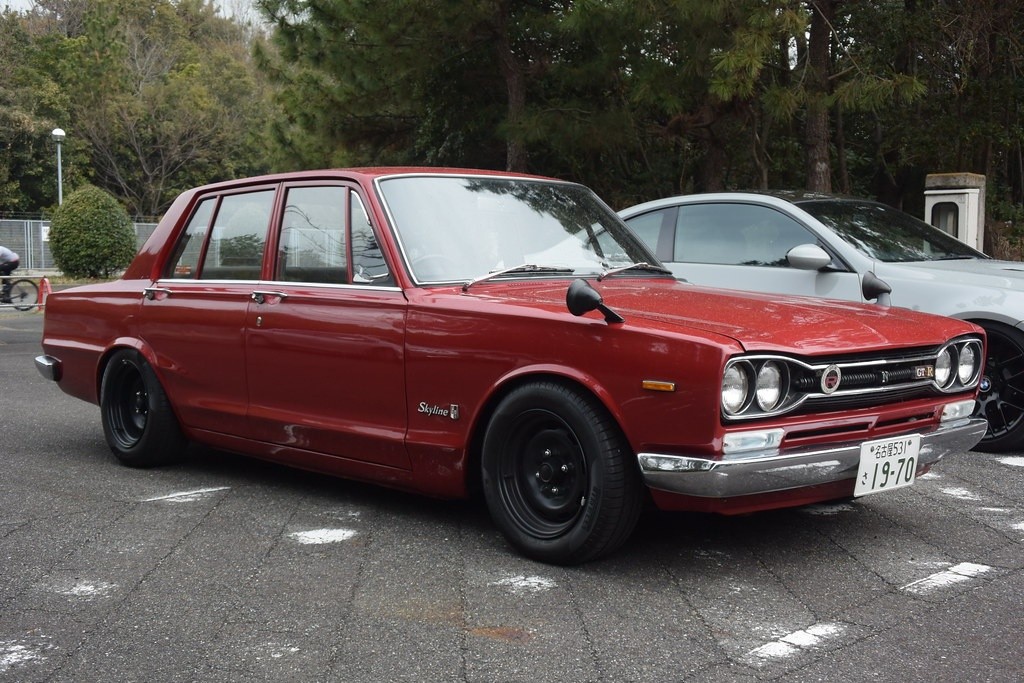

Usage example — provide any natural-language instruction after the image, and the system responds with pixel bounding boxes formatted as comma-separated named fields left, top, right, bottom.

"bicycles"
left=0, top=269, right=40, bottom=311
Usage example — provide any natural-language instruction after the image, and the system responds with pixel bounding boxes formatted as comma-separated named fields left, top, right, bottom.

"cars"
left=516, top=190, right=1023, bottom=455
left=34, top=165, right=991, bottom=564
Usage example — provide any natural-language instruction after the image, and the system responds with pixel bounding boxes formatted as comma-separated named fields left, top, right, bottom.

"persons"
left=0, top=245, right=20, bottom=303
